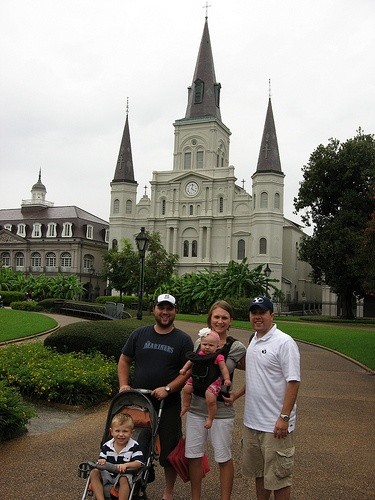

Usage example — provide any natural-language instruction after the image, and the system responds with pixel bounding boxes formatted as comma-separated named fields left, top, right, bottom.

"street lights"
left=264, top=264, right=272, bottom=297
left=87, top=265, right=95, bottom=302
left=133, top=227, right=150, bottom=320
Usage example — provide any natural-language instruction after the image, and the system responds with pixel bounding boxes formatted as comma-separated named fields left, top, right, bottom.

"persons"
left=183, top=301, right=247, bottom=500
left=241, top=295, right=301, bottom=500
left=89, top=412, right=146, bottom=500
left=179, top=327, right=232, bottom=429
left=118, top=294, right=195, bottom=500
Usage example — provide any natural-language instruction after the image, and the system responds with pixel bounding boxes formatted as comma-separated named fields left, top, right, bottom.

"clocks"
left=185, top=181, right=199, bottom=196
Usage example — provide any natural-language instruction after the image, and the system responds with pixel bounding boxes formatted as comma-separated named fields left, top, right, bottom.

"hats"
left=154, top=294, right=176, bottom=308
left=249, top=297, right=273, bottom=311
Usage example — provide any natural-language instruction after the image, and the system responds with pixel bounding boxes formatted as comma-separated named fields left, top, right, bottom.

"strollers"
left=76, top=388, right=165, bottom=500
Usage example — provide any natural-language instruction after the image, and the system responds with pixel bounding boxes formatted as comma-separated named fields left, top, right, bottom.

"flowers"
left=198, top=327, right=211, bottom=337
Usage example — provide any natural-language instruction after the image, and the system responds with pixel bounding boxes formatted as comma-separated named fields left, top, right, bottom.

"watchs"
left=280, top=414, right=291, bottom=422
left=164, top=385, right=172, bottom=395
